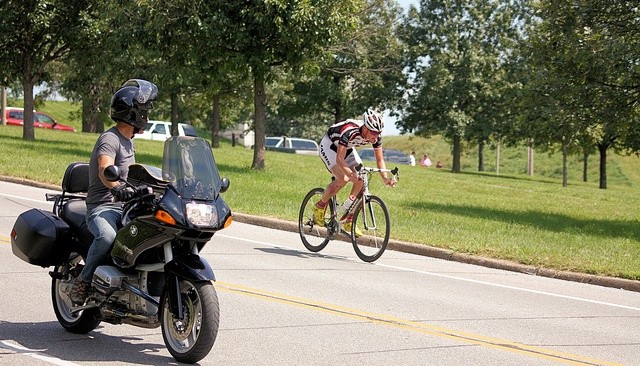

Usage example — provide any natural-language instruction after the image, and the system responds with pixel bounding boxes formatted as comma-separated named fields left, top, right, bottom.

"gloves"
left=109, top=184, right=136, bottom=202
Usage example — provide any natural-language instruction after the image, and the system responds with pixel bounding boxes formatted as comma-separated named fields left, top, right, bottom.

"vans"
left=265, top=136, right=320, bottom=157
left=359, top=147, right=409, bottom=165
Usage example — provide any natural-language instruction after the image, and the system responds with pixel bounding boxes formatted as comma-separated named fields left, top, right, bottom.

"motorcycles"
left=11, top=133, right=233, bottom=365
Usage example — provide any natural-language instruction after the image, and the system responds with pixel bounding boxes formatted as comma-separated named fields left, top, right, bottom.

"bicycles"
left=298, top=162, right=400, bottom=263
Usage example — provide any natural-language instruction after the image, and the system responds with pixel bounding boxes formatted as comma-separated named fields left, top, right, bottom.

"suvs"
left=134, top=120, right=197, bottom=142
left=0, top=105, right=78, bottom=132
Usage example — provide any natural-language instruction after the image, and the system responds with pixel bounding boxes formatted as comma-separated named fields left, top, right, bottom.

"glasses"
left=364, top=124, right=382, bottom=135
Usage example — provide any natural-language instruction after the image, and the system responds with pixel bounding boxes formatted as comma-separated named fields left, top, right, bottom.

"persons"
left=70, top=79, right=159, bottom=305
left=408, top=151, right=416, bottom=167
left=436, top=160, right=444, bottom=168
left=419, top=154, right=431, bottom=168
left=312, top=108, right=396, bottom=238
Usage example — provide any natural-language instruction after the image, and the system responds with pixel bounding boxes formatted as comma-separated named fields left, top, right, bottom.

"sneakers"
left=311, top=203, right=327, bottom=227
left=69, top=277, right=92, bottom=306
left=341, top=222, right=363, bottom=238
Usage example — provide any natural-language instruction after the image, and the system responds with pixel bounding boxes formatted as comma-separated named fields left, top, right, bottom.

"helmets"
left=110, top=78, right=158, bottom=131
left=363, top=109, right=384, bottom=133
